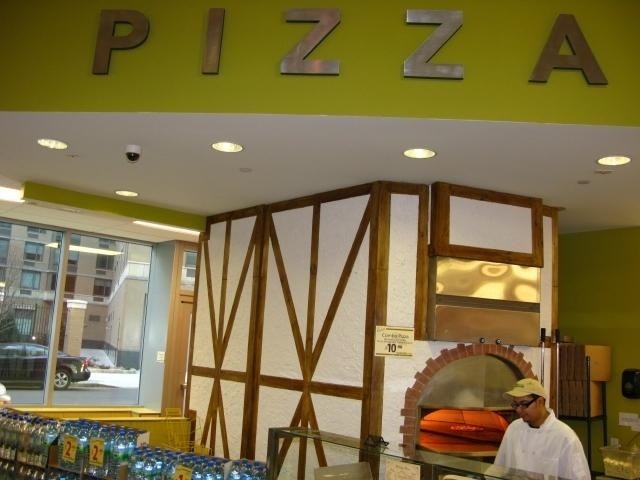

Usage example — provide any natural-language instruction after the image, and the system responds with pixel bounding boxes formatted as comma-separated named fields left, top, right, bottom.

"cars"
left=0, top=342, right=90, bottom=390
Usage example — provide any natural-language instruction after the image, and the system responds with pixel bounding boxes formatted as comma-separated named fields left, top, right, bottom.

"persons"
left=491, top=376, right=593, bottom=480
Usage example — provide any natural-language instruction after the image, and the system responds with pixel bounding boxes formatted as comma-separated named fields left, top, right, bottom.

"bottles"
left=0, top=409, right=268, bottom=480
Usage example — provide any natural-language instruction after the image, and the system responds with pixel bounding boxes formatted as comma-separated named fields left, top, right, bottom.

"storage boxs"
left=558, top=343, right=613, bottom=417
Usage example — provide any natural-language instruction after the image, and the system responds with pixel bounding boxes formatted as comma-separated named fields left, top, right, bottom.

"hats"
left=501, top=377, right=547, bottom=401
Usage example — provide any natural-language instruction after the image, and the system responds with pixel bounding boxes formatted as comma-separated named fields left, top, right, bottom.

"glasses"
left=511, top=395, right=540, bottom=409
